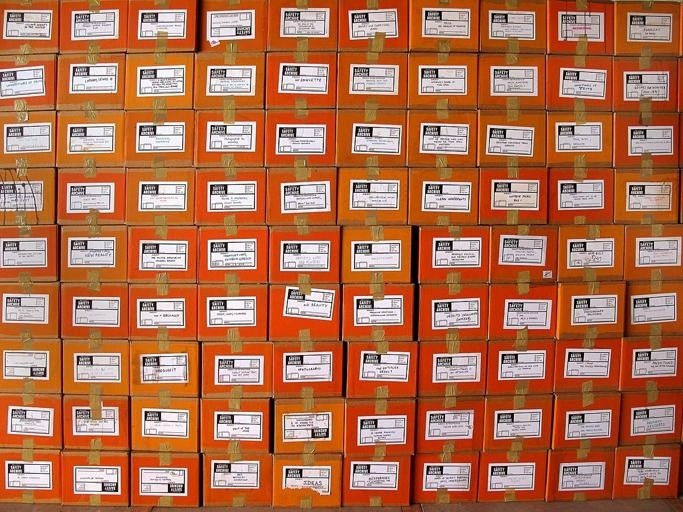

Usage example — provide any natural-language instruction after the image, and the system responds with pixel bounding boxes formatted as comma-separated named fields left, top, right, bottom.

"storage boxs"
left=0, top=449, right=61, bottom=505
left=128, top=284, right=197, bottom=341
left=342, top=283, right=415, bottom=340
left=547, top=111, right=613, bottom=167
left=408, top=110, right=478, bottom=168
left=414, top=453, right=479, bottom=502
left=340, top=1, right=408, bottom=53
left=418, top=226, right=490, bottom=283
left=1, top=1, right=59, bottom=49
left=64, top=395, right=130, bottom=450
left=554, top=392, right=619, bottom=449
left=269, top=227, right=341, bottom=284
left=337, top=109, right=407, bottom=167
left=126, top=169, right=195, bottom=225
left=614, top=169, right=679, bottom=224
left=418, top=341, right=488, bottom=396
left=61, top=451, right=131, bottom=505
left=198, top=226, right=268, bottom=283
left=127, top=1, right=197, bottom=52
left=347, top=342, right=417, bottom=399
left=479, top=167, right=548, bottom=225
left=275, top=342, right=344, bottom=398
left=125, top=110, right=194, bottom=167
left=202, top=1, right=268, bottom=52
left=344, top=401, right=415, bottom=458
left=202, top=454, right=273, bottom=505
left=196, top=54, right=265, bottom=108
left=0, top=340, right=62, bottom=391
left=622, top=338, right=683, bottom=390
left=487, top=396, right=553, bottom=449
left=623, top=390, right=682, bottom=443
left=0, top=227, right=58, bottom=282
left=342, top=227, right=412, bottom=283
left=418, top=283, right=490, bottom=340
left=197, top=284, right=267, bottom=341
left=63, top=340, right=129, bottom=396
left=549, top=168, right=614, bottom=224
left=59, top=54, right=124, bottom=109
left=489, top=283, right=558, bottom=340
left=614, top=445, right=680, bottom=500
left=130, top=452, right=200, bottom=506
left=409, top=51, right=478, bottom=110
left=130, top=341, right=200, bottom=397
left=546, top=56, right=612, bottom=111
left=409, top=1, right=478, bottom=51
left=199, top=399, right=271, bottom=453
left=127, top=227, right=197, bottom=284
left=60, top=226, right=126, bottom=283
left=266, top=168, right=337, bottom=225
left=490, top=225, right=558, bottom=282
left=0, top=282, right=60, bottom=339
left=0, top=55, right=56, bottom=111
left=194, top=110, right=265, bottom=167
left=418, top=398, right=485, bottom=451
left=267, top=53, right=336, bottom=109
left=60, top=1, right=126, bottom=52
left=0, top=168, right=56, bottom=225
left=126, top=55, right=193, bottom=109
left=408, top=169, right=479, bottom=225
left=555, top=340, right=620, bottom=389
left=549, top=449, right=616, bottom=500
left=628, top=281, right=683, bottom=336
left=550, top=2, right=614, bottom=56
left=613, top=112, right=680, bottom=168
left=480, top=53, right=545, bottom=110
left=265, top=108, right=336, bottom=168
left=558, top=225, right=625, bottom=281
left=268, top=1, right=338, bottom=50
left=613, top=57, right=678, bottom=111
left=344, top=458, right=411, bottom=507
left=614, top=3, right=681, bottom=56
left=202, top=342, right=273, bottom=398
left=274, top=399, right=346, bottom=452
left=482, top=452, right=549, bottom=503
left=130, top=396, right=200, bottom=453
left=477, top=111, right=547, bottom=166
left=60, top=284, right=129, bottom=340
left=57, top=111, right=125, bottom=167
left=489, top=342, right=556, bottom=393
left=0, top=393, right=62, bottom=449
left=482, top=1, right=546, bottom=52
left=195, top=169, right=266, bottom=224
left=0, top=112, right=56, bottom=168
left=557, top=282, right=627, bottom=338
left=268, top=286, right=342, bottom=341
left=57, top=169, right=126, bottom=226
left=337, top=168, right=408, bottom=226
left=624, top=224, right=683, bottom=281
left=338, top=52, right=407, bottom=109
left=273, top=453, right=342, bottom=508
